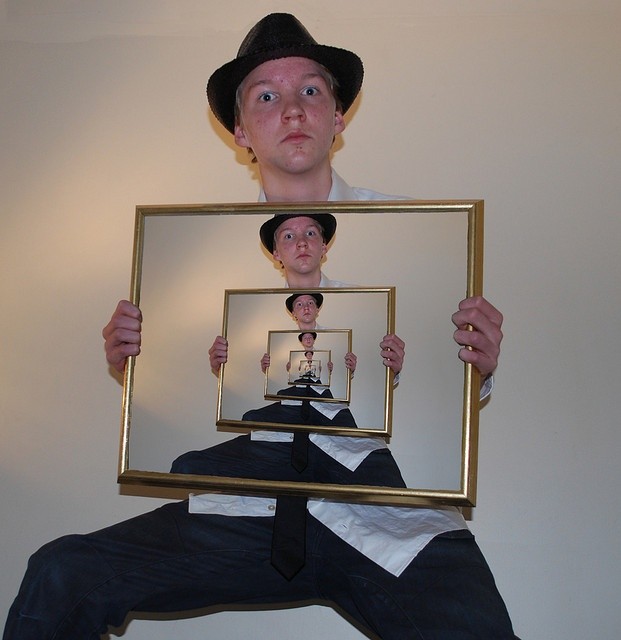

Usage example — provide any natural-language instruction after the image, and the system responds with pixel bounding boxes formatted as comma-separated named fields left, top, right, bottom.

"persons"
left=294, top=351, right=322, bottom=384
left=3, top=13, right=518, bottom=640
left=241, top=294, right=358, bottom=429
left=303, top=360, right=316, bottom=377
left=307, top=365, right=314, bottom=372
left=167, top=213, right=409, bottom=491
left=277, top=332, right=334, bottom=398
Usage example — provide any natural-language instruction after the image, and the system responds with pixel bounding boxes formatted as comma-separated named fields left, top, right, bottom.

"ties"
left=301, top=400, right=309, bottom=422
left=270, top=495, right=307, bottom=581
left=291, top=433, right=309, bottom=474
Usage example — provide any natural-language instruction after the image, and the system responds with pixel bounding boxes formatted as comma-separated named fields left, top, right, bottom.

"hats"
left=286, top=294, right=322, bottom=313
left=298, top=332, right=316, bottom=342
left=207, top=13, right=364, bottom=135
left=260, top=214, right=336, bottom=256
left=304, top=350, right=313, bottom=356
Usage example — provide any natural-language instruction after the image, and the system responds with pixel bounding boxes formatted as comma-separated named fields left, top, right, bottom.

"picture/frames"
left=288, top=350, right=331, bottom=387
left=216, top=286, right=396, bottom=437
left=305, top=365, right=316, bottom=374
left=118, top=202, right=484, bottom=508
left=299, top=360, right=321, bottom=378
left=264, top=329, right=353, bottom=404
